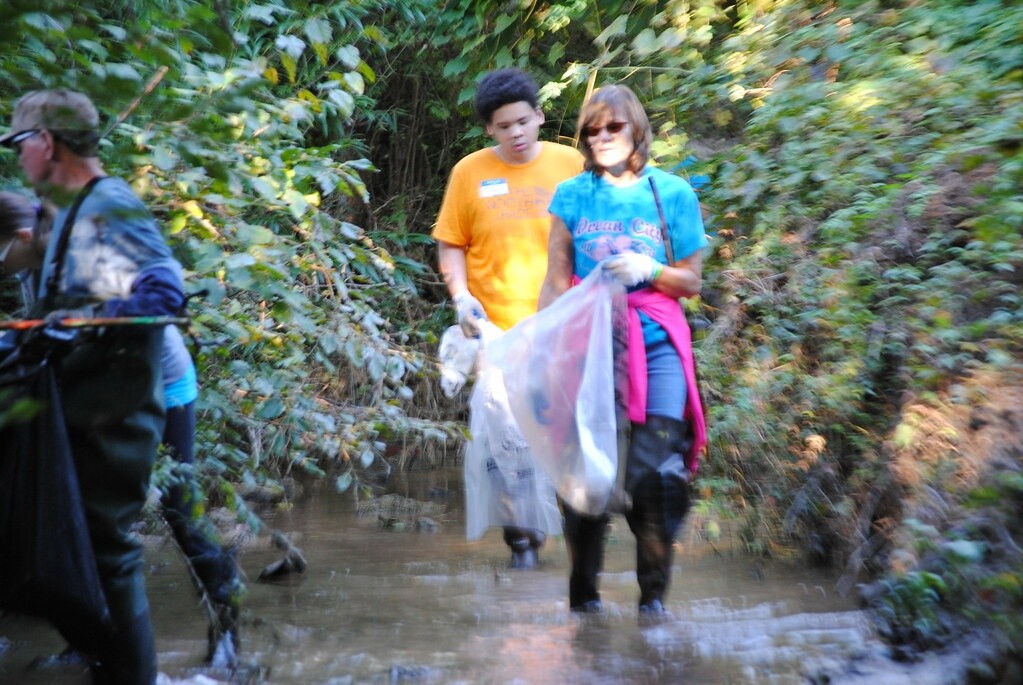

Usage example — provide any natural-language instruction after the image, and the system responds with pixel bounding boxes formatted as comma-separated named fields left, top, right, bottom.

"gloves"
left=606, top=252, right=663, bottom=287
left=452, top=290, right=488, bottom=339
left=21, top=303, right=103, bottom=364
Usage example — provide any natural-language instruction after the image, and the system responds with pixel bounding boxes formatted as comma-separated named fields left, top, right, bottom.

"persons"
left=434, top=67, right=588, bottom=570
left=534, top=83, right=707, bottom=620
left=0, top=84, right=245, bottom=677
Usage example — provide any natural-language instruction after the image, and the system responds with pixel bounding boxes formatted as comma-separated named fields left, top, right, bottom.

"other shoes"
left=185, top=579, right=247, bottom=664
left=502, top=526, right=545, bottom=570
left=29, top=649, right=91, bottom=685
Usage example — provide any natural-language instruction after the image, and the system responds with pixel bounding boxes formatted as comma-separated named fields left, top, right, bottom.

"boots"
left=628, top=472, right=687, bottom=625
left=554, top=493, right=611, bottom=612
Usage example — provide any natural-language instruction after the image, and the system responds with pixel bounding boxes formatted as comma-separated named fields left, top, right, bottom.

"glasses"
left=581, top=121, right=630, bottom=136
left=1, top=236, right=16, bottom=276
left=11, top=131, right=38, bottom=155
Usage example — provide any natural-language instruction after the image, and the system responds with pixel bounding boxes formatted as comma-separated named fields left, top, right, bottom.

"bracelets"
left=451, top=290, right=470, bottom=302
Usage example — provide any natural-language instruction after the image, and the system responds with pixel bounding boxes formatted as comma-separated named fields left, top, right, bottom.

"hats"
left=0, top=87, right=100, bottom=141
